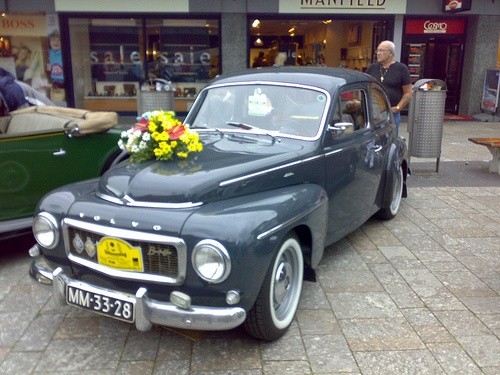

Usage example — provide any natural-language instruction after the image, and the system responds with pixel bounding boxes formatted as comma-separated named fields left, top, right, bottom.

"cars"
left=27, top=67, right=411, bottom=343
left=0, top=105, right=130, bottom=242
left=0, top=67, right=55, bottom=116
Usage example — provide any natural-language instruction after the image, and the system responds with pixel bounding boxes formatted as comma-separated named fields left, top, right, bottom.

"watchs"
left=395, top=105, right=401, bottom=111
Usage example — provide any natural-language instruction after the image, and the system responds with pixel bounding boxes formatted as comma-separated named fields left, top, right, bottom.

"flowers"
left=118, top=110, right=203, bottom=163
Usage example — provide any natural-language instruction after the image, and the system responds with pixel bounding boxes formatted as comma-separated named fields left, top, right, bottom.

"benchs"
left=5, top=111, right=77, bottom=135
left=468, top=138, right=500, bottom=175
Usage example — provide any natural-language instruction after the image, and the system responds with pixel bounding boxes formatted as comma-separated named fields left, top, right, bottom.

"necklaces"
left=380, top=61, right=395, bottom=83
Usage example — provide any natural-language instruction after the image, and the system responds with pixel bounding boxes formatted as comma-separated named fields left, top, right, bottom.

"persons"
left=11, top=47, right=39, bottom=85
left=365, top=40, right=414, bottom=132
left=0, top=66, right=27, bottom=113
left=254, top=51, right=327, bottom=69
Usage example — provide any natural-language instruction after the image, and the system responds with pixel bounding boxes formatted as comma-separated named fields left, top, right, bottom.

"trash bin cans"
left=407, top=78, right=448, bottom=158
left=137, top=78, right=174, bottom=115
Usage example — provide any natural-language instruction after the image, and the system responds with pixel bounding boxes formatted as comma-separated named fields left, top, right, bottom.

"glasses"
left=374, top=49, right=391, bottom=54
